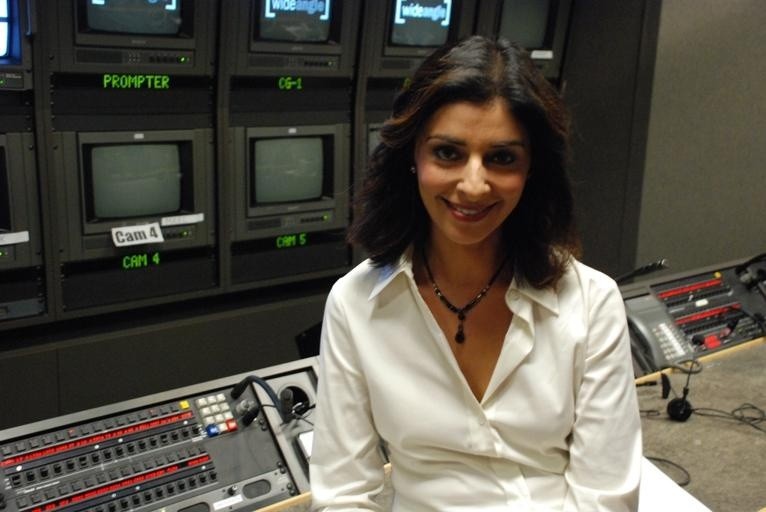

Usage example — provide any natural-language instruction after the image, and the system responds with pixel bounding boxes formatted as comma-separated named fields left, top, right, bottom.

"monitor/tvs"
left=73, top=0, right=198, bottom=50
left=0, top=0, right=32, bottom=71
left=246, top=123, right=344, bottom=217
left=77, top=129, right=207, bottom=235
left=249, top=0, right=343, bottom=56
left=474, top=0, right=570, bottom=81
left=382, top=0, right=462, bottom=58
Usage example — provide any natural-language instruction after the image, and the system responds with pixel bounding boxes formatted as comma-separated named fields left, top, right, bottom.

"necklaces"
left=418, top=247, right=509, bottom=346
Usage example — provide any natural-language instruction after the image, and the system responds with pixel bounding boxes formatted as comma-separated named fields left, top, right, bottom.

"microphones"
left=610, top=258, right=669, bottom=285
left=667, top=335, right=705, bottom=421
left=230, top=374, right=292, bottom=424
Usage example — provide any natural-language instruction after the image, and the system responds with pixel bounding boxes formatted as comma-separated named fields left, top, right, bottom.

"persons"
left=303, top=32, right=644, bottom=512
